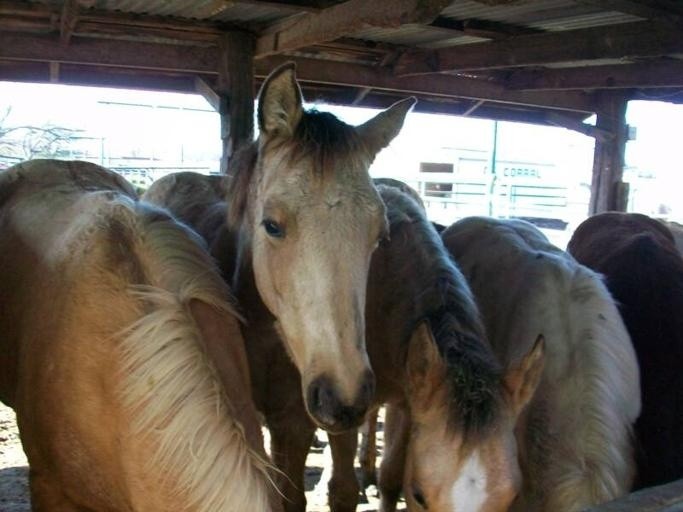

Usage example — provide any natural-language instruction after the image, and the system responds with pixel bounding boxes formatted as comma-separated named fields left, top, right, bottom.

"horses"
left=444, top=214, right=641, bottom=512
left=0, top=156, right=284, bottom=512
left=560, top=211, right=683, bottom=493
left=138, top=59, right=418, bottom=512
left=267, top=176, right=548, bottom=512
